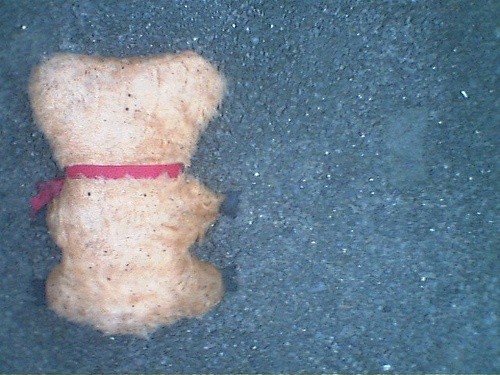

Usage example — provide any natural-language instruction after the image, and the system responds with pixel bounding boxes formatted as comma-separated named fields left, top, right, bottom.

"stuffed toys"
left=29, top=51, right=230, bottom=334
